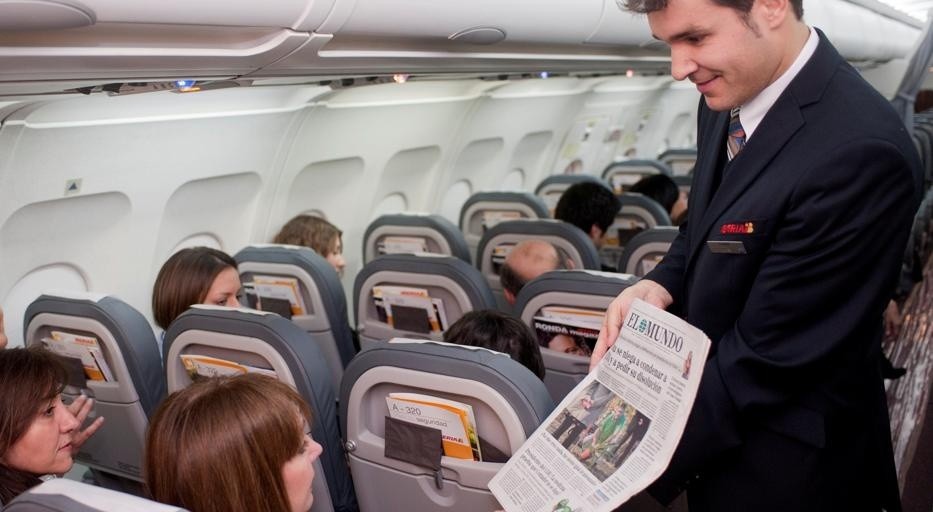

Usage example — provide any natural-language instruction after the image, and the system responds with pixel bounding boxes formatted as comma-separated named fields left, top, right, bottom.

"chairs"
left=164, top=304, right=360, bottom=512
left=534, top=174, right=613, bottom=218
left=600, top=158, right=666, bottom=194
left=913, top=106, right=933, bottom=183
left=23, top=292, right=165, bottom=496
left=457, top=190, right=553, bottom=268
left=512, top=269, right=644, bottom=412
left=4, top=479, right=187, bottom=512
left=226, top=242, right=356, bottom=407
left=617, top=227, right=680, bottom=277
left=657, top=148, right=698, bottom=177
left=363, top=214, right=473, bottom=269
left=668, top=176, right=690, bottom=193
left=340, top=338, right=558, bottom=512
left=352, top=252, right=497, bottom=355
left=475, top=220, right=603, bottom=317
left=598, top=193, right=673, bottom=271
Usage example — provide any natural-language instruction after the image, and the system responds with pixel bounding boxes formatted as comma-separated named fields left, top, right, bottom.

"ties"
left=722, top=103, right=745, bottom=158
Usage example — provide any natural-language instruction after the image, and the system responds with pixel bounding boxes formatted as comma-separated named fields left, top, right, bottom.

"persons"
left=272, top=214, right=361, bottom=355
left=143, top=373, right=323, bottom=511
left=443, top=310, right=546, bottom=381
left=1, top=346, right=105, bottom=511
left=554, top=182, right=623, bottom=274
left=152, top=245, right=245, bottom=330
left=589, top=0, right=925, bottom=512
left=913, top=88, right=931, bottom=177
left=628, top=173, right=689, bottom=228
left=501, top=240, right=576, bottom=308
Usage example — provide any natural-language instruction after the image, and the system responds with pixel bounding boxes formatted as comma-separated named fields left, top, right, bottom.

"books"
left=369, top=282, right=449, bottom=337
left=480, top=209, right=520, bottom=233
left=384, top=390, right=482, bottom=462
left=35, top=328, right=113, bottom=383
left=534, top=306, right=608, bottom=355
left=376, top=236, right=428, bottom=256
left=243, top=271, right=306, bottom=318
